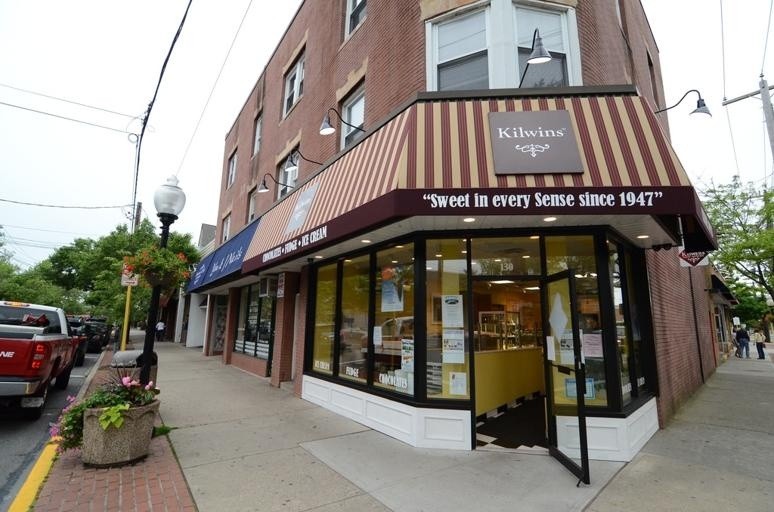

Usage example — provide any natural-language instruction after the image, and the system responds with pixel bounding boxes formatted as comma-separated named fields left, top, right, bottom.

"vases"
left=82, top=401, right=158, bottom=468
left=145, top=274, right=178, bottom=289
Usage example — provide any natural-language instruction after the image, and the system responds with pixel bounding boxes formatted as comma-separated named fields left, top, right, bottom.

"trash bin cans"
left=111, top=350, right=157, bottom=391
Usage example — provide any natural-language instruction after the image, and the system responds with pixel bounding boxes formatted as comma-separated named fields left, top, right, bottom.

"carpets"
left=473, top=385, right=552, bottom=449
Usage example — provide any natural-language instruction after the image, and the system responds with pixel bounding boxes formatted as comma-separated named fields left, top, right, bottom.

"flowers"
left=48, top=377, right=161, bottom=453
left=122, top=248, right=192, bottom=282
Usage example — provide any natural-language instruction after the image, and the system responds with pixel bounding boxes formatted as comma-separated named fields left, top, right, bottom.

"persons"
left=154, top=319, right=165, bottom=342
left=731, top=326, right=744, bottom=359
left=110, top=325, right=119, bottom=353
left=752, top=329, right=766, bottom=360
left=734, top=324, right=752, bottom=360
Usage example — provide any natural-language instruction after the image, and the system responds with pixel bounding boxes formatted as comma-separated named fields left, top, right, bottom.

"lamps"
left=518, top=30, right=552, bottom=88
left=316, top=108, right=366, bottom=137
left=656, top=89, right=712, bottom=121
left=256, top=148, right=323, bottom=195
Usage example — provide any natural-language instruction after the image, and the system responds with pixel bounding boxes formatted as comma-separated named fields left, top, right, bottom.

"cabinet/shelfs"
left=478, top=311, right=521, bottom=352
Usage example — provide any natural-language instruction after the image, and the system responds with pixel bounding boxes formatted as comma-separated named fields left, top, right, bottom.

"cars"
left=0, top=296, right=117, bottom=422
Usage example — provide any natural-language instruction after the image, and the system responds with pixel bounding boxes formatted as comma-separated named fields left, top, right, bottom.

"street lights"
left=137, top=173, right=186, bottom=392
